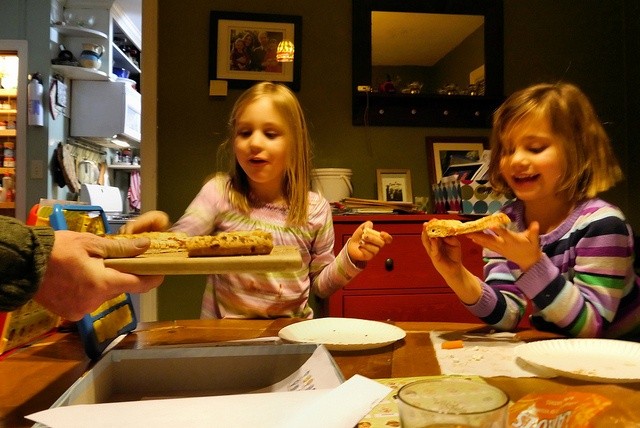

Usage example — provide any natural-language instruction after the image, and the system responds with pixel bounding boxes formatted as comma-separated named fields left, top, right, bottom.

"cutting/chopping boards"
left=104, top=244, right=302, bottom=275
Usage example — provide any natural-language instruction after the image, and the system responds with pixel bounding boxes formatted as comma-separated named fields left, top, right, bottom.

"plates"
left=513, top=337, right=640, bottom=384
left=277, top=317, right=407, bottom=351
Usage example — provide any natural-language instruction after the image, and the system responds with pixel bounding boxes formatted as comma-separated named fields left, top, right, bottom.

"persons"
left=112, top=79, right=393, bottom=322
left=0, top=215, right=167, bottom=324
left=230, top=25, right=284, bottom=75
left=422, top=83, right=635, bottom=337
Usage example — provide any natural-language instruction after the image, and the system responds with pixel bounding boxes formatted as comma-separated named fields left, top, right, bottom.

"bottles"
left=3, top=172, right=13, bottom=202
left=123, top=148, right=132, bottom=165
left=28, top=72, right=43, bottom=127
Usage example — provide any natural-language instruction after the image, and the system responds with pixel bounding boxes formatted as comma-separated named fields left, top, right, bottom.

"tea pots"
left=79, top=42, right=104, bottom=69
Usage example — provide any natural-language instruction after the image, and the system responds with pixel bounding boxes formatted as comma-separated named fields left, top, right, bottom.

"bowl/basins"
left=62, top=9, right=97, bottom=28
left=113, top=67, right=131, bottom=79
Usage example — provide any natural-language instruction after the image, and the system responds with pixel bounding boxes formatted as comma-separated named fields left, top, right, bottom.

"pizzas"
left=105, top=230, right=190, bottom=253
left=186, top=230, right=273, bottom=256
left=427, top=213, right=511, bottom=239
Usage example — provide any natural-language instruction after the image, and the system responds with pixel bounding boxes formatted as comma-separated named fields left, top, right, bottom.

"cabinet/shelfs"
left=48, top=4, right=141, bottom=90
left=327, top=214, right=491, bottom=322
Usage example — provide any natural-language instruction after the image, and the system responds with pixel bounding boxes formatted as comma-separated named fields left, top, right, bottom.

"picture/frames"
left=206, top=8, right=304, bottom=93
left=425, top=134, right=489, bottom=201
left=376, top=168, right=414, bottom=206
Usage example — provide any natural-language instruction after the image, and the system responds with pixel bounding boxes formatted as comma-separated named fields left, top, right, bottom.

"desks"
left=0, top=314, right=640, bottom=428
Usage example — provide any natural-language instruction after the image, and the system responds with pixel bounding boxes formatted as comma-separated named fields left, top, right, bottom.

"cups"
left=397, top=378, right=510, bottom=428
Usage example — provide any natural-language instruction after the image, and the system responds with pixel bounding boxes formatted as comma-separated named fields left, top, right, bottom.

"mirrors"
left=351, top=2, right=503, bottom=128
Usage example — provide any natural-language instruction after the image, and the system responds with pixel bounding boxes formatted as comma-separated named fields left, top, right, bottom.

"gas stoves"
left=103, top=213, right=140, bottom=222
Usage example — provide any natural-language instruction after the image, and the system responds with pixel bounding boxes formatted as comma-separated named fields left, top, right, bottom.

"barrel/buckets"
left=310, top=167, right=354, bottom=205
